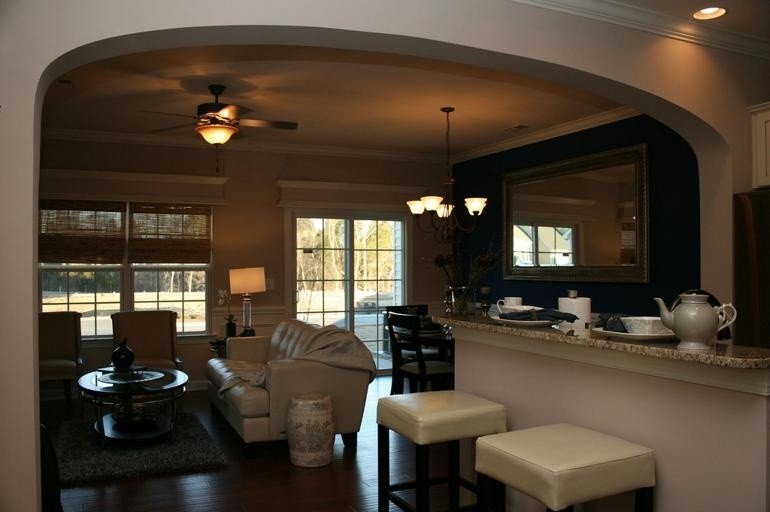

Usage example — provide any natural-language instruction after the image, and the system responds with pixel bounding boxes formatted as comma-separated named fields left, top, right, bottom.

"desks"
left=385, top=325, right=454, bottom=361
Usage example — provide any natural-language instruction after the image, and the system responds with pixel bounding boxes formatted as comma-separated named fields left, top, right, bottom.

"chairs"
left=387, top=305, right=427, bottom=350
left=38, top=312, right=84, bottom=415
left=388, top=311, right=454, bottom=394
left=111, top=310, right=183, bottom=411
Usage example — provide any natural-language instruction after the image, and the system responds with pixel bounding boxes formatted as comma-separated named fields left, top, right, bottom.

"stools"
left=475, top=423, right=656, bottom=512
left=378, top=390, right=508, bottom=512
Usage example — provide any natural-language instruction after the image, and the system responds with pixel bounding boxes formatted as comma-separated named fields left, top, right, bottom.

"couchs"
left=205, top=318, right=376, bottom=445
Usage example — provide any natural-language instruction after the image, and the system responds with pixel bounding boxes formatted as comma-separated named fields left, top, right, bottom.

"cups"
left=497, top=296, right=522, bottom=314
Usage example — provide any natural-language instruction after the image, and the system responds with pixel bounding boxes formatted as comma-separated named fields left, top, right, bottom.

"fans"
left=140, top=84, right=298, bottom=136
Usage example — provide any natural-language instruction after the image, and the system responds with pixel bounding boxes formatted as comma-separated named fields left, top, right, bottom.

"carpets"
left=52, top=412, right=229, bottom=488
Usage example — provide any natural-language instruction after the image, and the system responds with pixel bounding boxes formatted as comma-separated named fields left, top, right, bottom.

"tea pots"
left=653, top=294, right=737, bottom=349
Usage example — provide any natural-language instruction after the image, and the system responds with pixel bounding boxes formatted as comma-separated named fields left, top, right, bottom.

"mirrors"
left=502, top=143, right=650, bottom=284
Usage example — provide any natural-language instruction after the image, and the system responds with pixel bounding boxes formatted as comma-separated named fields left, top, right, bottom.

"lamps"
left=196, top=123, right=238, bottom=146
left=229, top=267, right=266, bottom=329
left=406, top=106, right=488, bottom=219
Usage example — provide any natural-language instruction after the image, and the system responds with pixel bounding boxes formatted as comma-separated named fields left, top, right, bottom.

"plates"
left=491, top=315, right=562, bottom=326
left=593, top=327, right=676, bottom=341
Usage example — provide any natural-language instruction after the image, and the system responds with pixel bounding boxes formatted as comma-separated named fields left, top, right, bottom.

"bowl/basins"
left=620, top=316, right=668, bottom=336
left=503, top=305, right=544, bottom=314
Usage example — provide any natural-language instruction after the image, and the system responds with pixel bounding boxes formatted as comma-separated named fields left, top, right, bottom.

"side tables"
left=208, top=340, right=226, bottom=357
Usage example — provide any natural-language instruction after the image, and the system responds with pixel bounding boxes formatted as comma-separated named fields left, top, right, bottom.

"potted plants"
left=434, top=250, right=497, bottom=316
left=226, top=311, right=236, bottom=337
